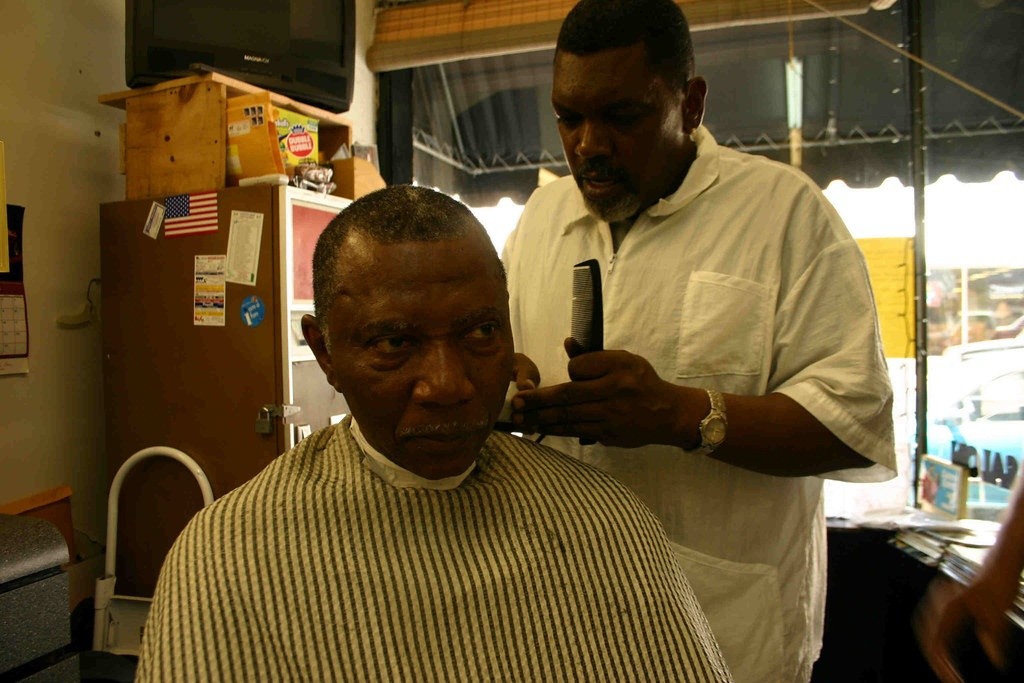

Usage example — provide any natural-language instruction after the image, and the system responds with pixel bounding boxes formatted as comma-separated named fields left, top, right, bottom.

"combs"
left=572, top=258, right=604, bottom=445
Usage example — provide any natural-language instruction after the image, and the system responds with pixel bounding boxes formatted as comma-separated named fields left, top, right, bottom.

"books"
left=888, top=529, right=1024, bottom=628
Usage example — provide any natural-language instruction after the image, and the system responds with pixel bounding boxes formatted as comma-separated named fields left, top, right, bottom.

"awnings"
left=412, top=0, right=1024, bottom=208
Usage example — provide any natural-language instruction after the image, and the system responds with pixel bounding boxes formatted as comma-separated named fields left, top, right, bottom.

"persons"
left=135, top=186, right=731, bottom=683
left=925, top=460, right=1024, bottom=683
left=500, top=0, right=899, bottom=683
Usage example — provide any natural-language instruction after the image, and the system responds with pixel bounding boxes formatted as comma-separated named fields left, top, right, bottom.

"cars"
left=915, top=342, right=1024, bottom=527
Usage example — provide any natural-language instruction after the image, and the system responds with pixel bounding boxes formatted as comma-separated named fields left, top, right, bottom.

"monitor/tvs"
left=124, top=0, right=356, bottom=115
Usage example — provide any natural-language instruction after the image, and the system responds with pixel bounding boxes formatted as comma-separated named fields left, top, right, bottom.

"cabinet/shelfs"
left=100, top=184, right=352, bottom=600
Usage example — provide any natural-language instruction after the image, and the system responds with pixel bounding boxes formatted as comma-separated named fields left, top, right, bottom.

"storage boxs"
left=271, top=105, right=320, bottom=169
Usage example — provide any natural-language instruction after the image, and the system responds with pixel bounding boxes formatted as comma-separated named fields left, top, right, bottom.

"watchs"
left=685, top=388, right=729, bottom=456
